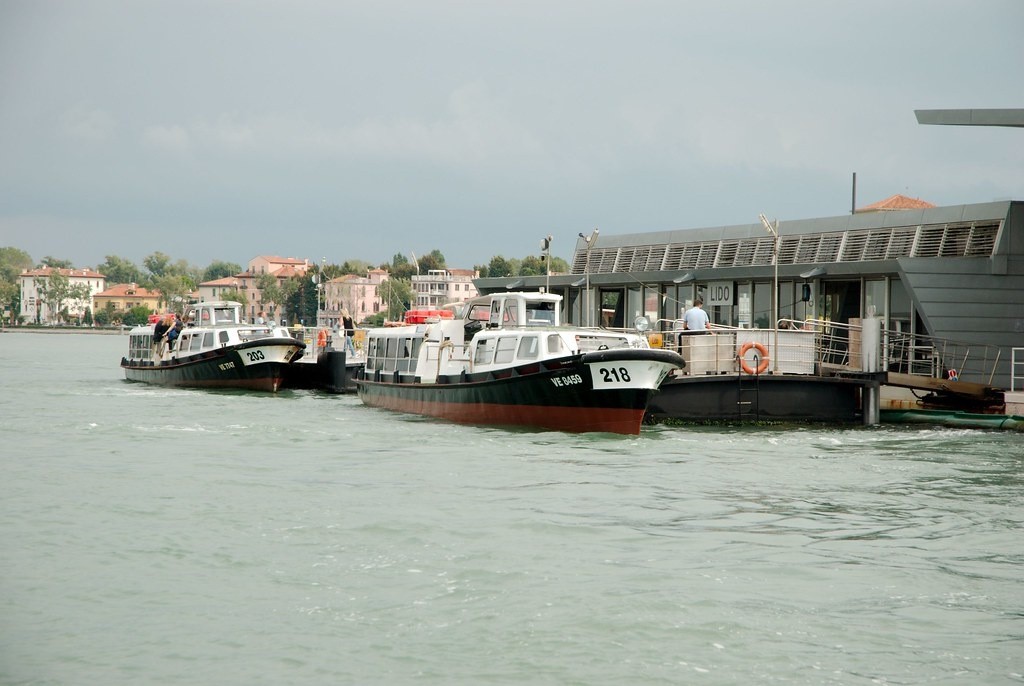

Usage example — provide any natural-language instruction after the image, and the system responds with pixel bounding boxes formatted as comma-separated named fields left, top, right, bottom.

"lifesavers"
left=344, top=336, right=355, bottom=355
left=384, top=320, right=404, bottom=326
left=316, top=330, right=327, bottom=347
left=738, top=342, right=769, bottom=373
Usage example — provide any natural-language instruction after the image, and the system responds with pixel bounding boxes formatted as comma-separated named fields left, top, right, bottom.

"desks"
left=681, top=335, right=734, bottom=375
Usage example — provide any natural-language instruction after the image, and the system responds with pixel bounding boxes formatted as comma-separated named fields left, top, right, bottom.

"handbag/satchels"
left=332, top=325, right=337, bottom=332
left=338, top=325, right=344, bottom=337
left=169, top=329, right=179, bottom=340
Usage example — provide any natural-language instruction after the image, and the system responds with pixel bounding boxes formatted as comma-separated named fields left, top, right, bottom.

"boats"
left=120, top=301, right=307, bottom=393
left=350, top=286, right=687, bottom=435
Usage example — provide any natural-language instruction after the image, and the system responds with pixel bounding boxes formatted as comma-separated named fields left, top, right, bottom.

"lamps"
left=802, top=278, right=811, bottom=302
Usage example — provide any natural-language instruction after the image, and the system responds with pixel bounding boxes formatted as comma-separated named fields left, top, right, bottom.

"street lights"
left=539, top=239, right=550, bottom=293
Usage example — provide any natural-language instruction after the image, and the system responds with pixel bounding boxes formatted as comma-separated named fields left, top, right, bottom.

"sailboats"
left=647, top=216, right=882, bottom=428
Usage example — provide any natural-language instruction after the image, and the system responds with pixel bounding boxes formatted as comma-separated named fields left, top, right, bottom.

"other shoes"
left=349, top=354, right=360, bottom=359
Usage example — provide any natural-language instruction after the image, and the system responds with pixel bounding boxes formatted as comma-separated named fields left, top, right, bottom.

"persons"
left=255, top=311, right=268, bottom=333
left=677, top=300, right=716, bottom=355
left=779, top=316, right=789, bottom=329
left=338, top=309, right=360, bottom=359
left=159, top=311, right=183, bottom=356
left=536, top=302, right=550, bottom=319
left=182, top=315, right=194, bottom=340
left=149, top=317, right=172, bottom=354
